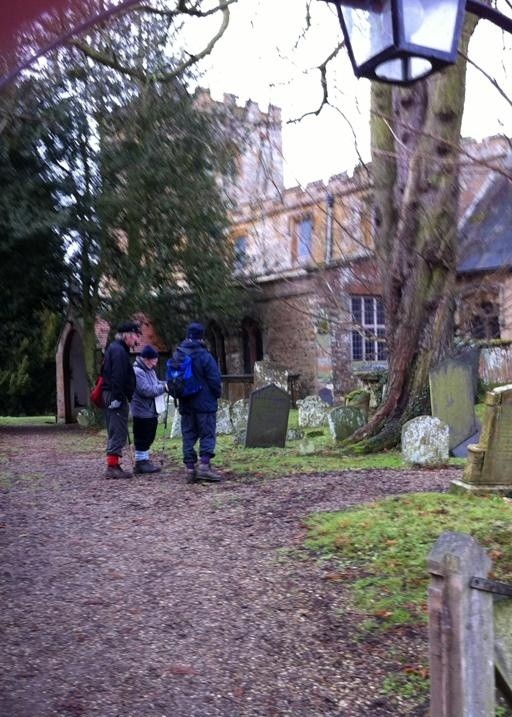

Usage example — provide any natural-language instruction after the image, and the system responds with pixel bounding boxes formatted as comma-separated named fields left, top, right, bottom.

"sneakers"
left=185, top=467, right=196, bottom=484
left=104, top=458, right=162, bottom=479
left=196, top=463, right=223, bottom=481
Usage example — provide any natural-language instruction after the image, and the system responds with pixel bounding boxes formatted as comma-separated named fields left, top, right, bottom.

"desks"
left=220, top=370, right=301, bottom=407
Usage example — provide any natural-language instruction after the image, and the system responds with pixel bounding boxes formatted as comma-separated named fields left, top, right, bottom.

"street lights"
left=318, top=1, right=511, bottom=89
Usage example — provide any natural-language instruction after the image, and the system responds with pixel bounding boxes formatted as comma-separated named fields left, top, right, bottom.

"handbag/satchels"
left=89, top=374, right=106, bottom=409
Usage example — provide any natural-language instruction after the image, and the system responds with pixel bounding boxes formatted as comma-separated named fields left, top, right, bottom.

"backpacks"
left=164, top=347, right=207, bottom=400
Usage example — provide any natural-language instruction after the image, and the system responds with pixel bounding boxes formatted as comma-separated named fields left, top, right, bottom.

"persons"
left=102, top=322, right=141, bottom=480
left=131, top=345, right=171, bottom=474
left=175, top=322, right=223, bottom=484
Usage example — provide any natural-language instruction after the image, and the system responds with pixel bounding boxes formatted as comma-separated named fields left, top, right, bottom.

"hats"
left=141, top=343, right=159, bottom=358
left=117, top=321, right=142, bottom=335
left=185, top=322, right=206, bottom=340
